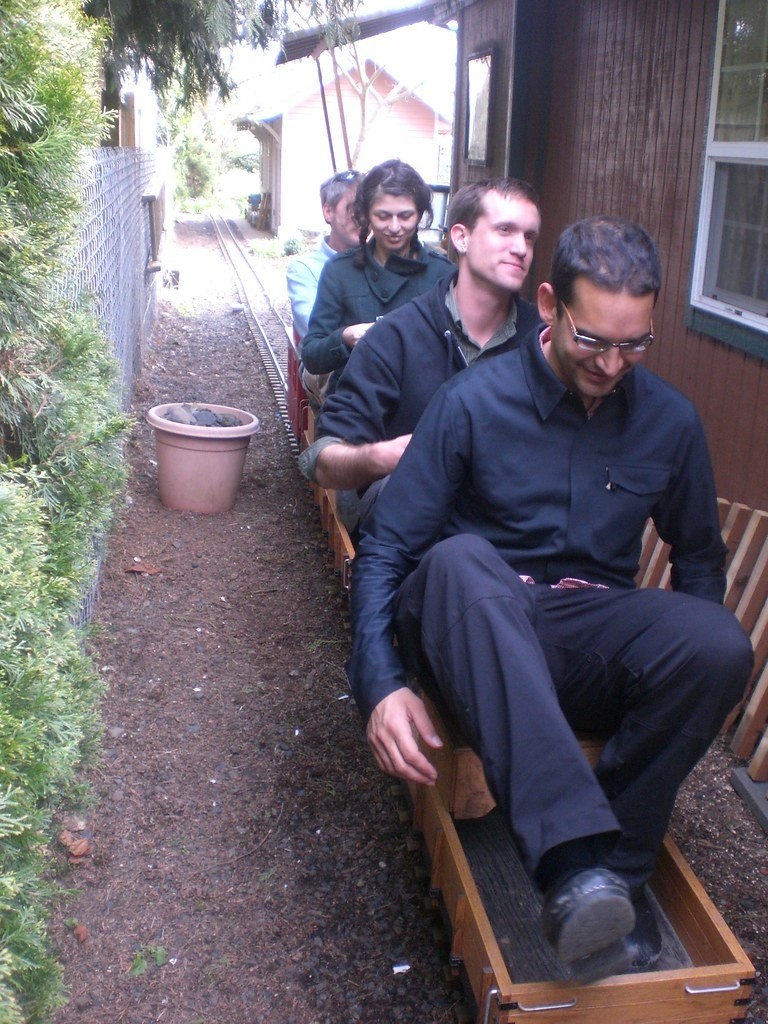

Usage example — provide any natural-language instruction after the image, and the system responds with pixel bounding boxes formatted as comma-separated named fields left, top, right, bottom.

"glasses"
left=335, top=171, right=359, bottom=182
left=559, top=300, right=655, bottom=354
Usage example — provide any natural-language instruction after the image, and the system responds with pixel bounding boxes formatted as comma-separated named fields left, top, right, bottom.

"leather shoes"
left=625, top=892, right=663, bottom=968
left=541, top=868, right=635, bottom=985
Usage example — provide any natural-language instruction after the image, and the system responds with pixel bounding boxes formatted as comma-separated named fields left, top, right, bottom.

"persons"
left=346, top=218, right=755, bottom=985
left=297, top=178, right=545, bottom=536
left=287, top=170, right=370, bottom=332
left=301, top=159, right=456, bottom=397
